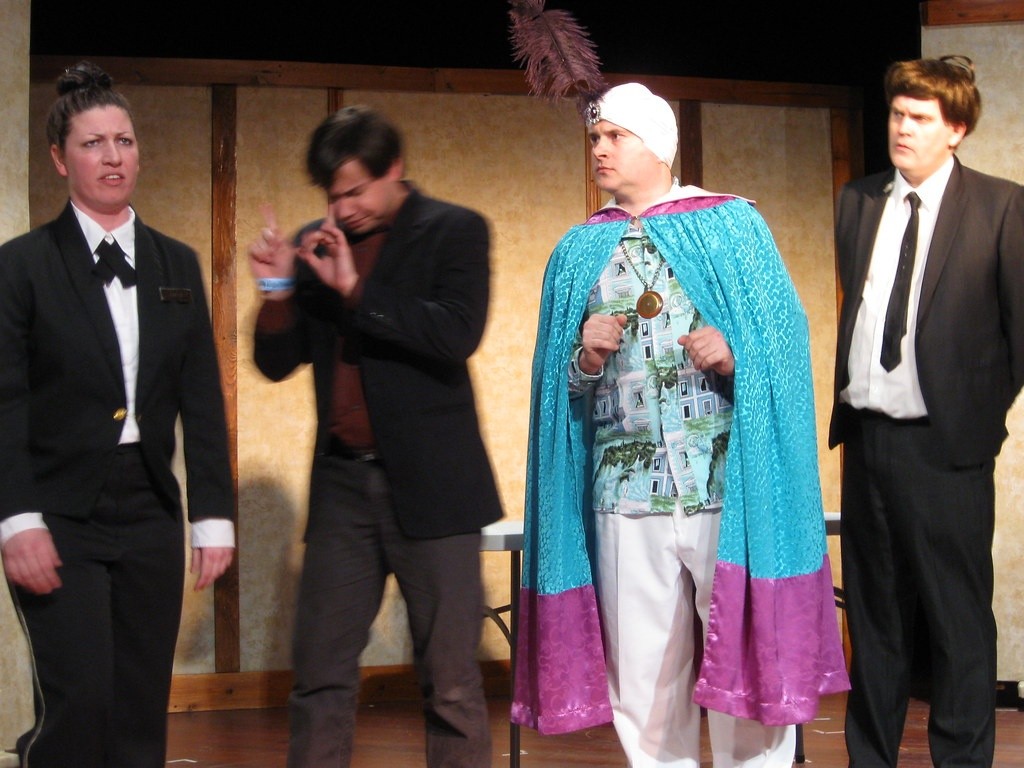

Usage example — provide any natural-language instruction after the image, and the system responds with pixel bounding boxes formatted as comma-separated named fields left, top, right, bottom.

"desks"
left=479, top=513, right=842, bottom=768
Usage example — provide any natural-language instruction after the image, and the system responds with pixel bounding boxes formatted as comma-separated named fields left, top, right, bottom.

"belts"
left=329, top=440, right=385, bottom=464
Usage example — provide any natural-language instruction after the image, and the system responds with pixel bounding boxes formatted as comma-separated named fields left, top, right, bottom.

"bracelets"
left=257, top=278, right=295, bottom=291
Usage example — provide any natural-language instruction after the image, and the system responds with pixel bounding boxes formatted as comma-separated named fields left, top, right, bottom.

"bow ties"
left=94, top=239, right=137, bottom=289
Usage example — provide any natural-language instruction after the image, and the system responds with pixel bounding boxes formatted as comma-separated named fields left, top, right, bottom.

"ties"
left=880, top=191, right=922, bottom=373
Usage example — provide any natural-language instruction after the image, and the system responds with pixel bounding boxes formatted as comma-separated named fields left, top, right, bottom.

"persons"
left=249, top=108, right=505, bottom=768
left=0, top=63, right=236, bottom=768
left=510, top=83, right=850, bottom=768
left=829, top=54, right=1024, bottom=768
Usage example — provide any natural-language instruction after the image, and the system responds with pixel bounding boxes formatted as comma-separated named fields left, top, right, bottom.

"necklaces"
left=619, top=239, right=666, bottom=319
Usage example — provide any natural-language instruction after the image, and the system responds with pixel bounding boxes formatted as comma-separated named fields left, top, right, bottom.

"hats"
left=585, top=81, right=679, bottom=170
